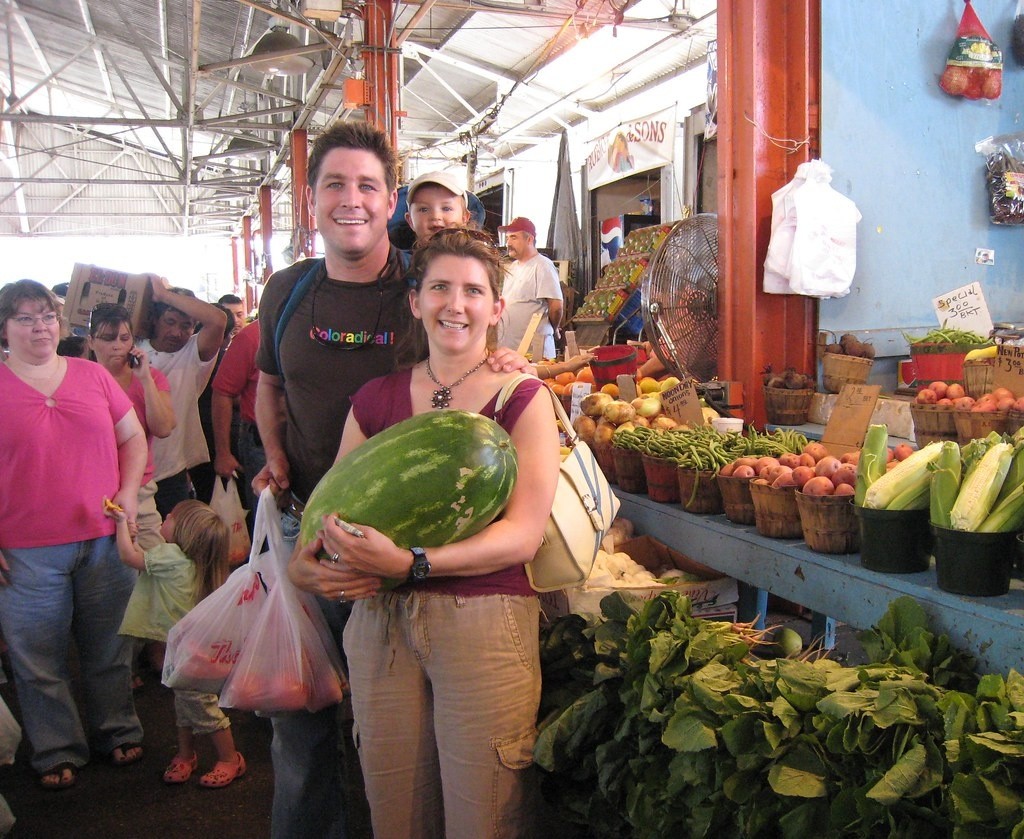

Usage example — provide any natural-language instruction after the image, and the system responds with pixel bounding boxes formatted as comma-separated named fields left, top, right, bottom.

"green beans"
left=611, top=421, right=814, bottom=508
left=901, top=319, right=995, bottom=347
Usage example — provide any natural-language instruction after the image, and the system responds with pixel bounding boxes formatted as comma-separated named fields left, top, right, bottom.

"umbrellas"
left=546, top=130, right=584, bottom=317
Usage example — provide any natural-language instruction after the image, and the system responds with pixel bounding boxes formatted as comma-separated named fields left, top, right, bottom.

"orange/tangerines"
left=541, top=366, right=680, bottom=397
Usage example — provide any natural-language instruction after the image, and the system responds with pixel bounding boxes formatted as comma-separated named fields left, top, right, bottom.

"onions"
left=573, top=392, right=720, bottom=444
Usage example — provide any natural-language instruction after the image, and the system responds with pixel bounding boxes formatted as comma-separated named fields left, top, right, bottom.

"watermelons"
left=300, top=409, right=518, bottom=592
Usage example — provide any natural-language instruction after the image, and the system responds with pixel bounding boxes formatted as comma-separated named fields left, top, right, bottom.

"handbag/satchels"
left=208, top=471, right=251, bottom=564
left=0, top=694, right=22, bottom=765
left=162, top=487, right=353, bottom=714
left=493, top=374, right=622, bottom=592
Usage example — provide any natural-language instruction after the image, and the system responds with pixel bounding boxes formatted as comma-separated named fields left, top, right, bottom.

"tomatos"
left=227, top=650, right=342, bottom=711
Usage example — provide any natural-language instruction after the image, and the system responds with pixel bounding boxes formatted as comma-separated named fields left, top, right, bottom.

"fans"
left=640, top=211, right=717, bottom=384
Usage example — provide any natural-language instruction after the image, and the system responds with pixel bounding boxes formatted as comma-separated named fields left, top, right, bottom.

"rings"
left=331, top=553, right=339, bottom=564
left=340, top=590, right=346, bottom=602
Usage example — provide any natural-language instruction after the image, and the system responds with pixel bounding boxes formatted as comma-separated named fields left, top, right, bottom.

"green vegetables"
left=535, top=590, right=1024, bottom=839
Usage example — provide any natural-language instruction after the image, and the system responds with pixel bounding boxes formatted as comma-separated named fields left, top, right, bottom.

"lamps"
left=223, top=89, right=271, bottom=160
left=250, top=8, right=318, bottom=76
left=210, top=166, right=238, bottom=214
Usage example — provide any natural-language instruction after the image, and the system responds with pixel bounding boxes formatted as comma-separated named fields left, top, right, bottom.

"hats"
left=466, top=190, right=486, bottom=227
left=404, top=169, right=467, bottom=214
left=496, top=217, right=536, bottom=238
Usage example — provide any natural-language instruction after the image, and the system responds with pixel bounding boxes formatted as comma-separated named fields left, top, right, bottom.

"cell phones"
left=129, top=353, right=139, bottom=370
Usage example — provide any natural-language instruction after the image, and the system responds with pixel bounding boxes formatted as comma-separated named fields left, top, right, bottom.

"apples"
left=577, top=229, right=668, bottom=316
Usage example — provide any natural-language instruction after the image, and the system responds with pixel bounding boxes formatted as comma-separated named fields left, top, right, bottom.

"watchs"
left=408, top=547, right=431, bottom=586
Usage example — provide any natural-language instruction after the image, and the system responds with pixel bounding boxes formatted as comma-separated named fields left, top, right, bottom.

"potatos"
left=720, top=442, right=913, bottom=495
left=607, top=517, right=634, bottom=545
left=915, top=381, right=1024, bottom=412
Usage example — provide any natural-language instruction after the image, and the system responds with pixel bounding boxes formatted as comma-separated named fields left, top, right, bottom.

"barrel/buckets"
left=554, top=343, right=1024, bottom=596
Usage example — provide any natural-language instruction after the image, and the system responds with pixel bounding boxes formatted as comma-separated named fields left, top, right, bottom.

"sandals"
left=163, top=749, right=197, bottom=783
left=202, top=751, right=246, bottom=787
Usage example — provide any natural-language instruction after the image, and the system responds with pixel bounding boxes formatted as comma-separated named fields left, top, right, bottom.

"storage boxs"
left=573, top=220, right=674, bottom=323
left=565, top=539, right=743, bottom=622
left=60, top=261, right=153, bottom=330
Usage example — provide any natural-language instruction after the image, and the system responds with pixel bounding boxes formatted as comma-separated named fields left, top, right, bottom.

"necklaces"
left=6, top=355, right=60, bottom=380
left=426, top=349, right=490, bottom=409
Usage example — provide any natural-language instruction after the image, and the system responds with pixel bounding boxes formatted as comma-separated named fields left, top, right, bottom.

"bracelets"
left=544, top=364, right=552, bottom=379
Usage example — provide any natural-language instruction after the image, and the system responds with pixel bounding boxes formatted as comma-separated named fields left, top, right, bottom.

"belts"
left=286, top=493, right=307, bottom=522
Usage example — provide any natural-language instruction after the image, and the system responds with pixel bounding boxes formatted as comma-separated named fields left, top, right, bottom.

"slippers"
left=40, top=761, right=76, bottom=788
left=111, top=740, right=143, bottom=766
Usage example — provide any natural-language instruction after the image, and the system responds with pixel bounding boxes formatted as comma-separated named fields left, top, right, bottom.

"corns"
left=855, top=425, right=1024, bottom=532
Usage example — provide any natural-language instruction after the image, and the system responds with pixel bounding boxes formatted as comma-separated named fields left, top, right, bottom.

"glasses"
left=6, top=314, right=61, bottom=328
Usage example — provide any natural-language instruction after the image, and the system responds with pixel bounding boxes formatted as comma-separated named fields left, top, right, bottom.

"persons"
left=497, top=217, right=563, bottom=362
left=251, top=122, right=538, bottom=839
left=532, top=353, right=598, bottom=379
left=102, top=495, right=246, bottom=786
left=404, top=172, right=470, bottom=247
left=0, top=273, right=269, bottom=790
left=289, top=234, right=560, bottom=838
